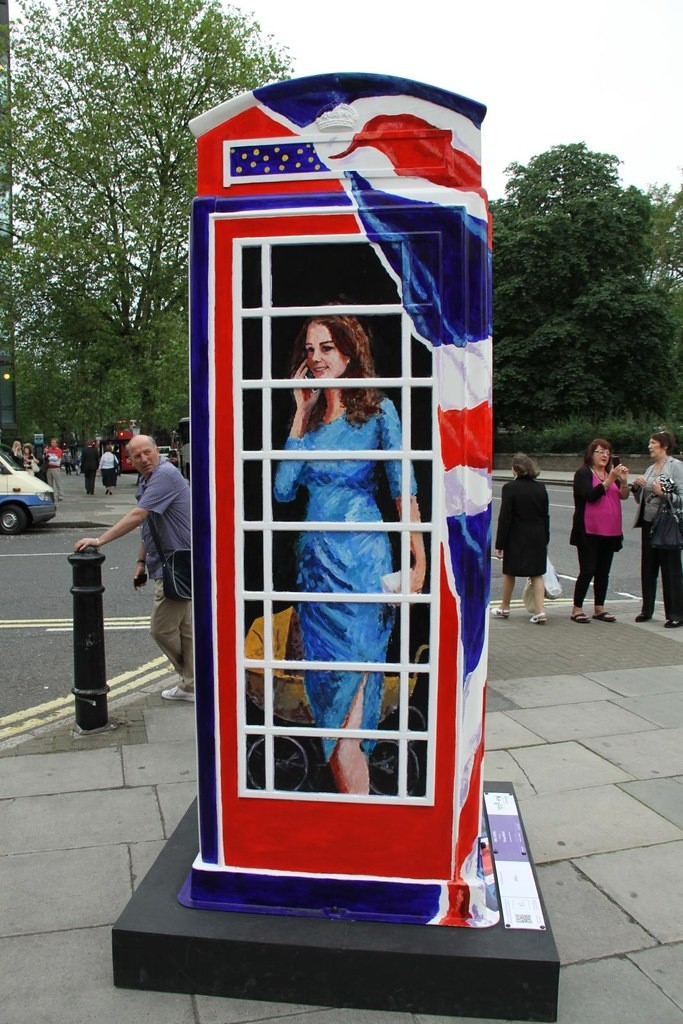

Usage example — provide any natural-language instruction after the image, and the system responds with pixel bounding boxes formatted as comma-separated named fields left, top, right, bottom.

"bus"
left=104, top=420, right=149, bottom=472
left=172, top=417, right=191, bottom=479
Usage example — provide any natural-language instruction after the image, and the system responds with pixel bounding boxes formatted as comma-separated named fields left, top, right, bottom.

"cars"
left=0, top=450, right=57, bottom=534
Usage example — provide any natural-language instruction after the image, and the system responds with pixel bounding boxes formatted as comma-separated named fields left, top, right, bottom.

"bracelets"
left=96, top=538, right=101, bottom=546
left=137, top=560, right=146, bottom=563
left=621, top=484, right=628, bottom=488
left=603, top=481, right=609, bottom=489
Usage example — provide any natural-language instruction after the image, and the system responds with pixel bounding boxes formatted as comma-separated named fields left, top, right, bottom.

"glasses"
left=594, top=450, right=610, bottom=456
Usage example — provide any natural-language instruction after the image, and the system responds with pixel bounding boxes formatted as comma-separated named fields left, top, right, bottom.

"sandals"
left=491, top=608, right=510, bottom=620
left=530, top=613, right=547, bottom=625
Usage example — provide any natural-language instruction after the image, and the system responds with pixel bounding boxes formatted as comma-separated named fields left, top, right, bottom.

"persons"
left=490, top=453, right=550, bottom=625
left=569, top=438, right=629, bottom=624
left=73, top=435, right=196, bottom=703
left=631, top=431, right=683, bottom=628
left=9, top=436, right=178, bottom=500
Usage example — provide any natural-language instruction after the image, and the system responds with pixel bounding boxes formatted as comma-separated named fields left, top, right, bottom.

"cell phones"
left=134, top=574, right=148, bottom=586
left=612, top=456, right=620, bottom=469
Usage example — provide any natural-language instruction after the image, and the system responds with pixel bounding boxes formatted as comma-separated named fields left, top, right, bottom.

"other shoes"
left=105, top=489, right=112, bottom=495
left=58, top=495, right=62, bottom=501
left=67, top=472, right=71, bottom=475
left=636, top=614, right=652, bottom=622
left=665, top=619, right=683, bottom=628
left=87, top=490, right=94, bottom=494
left=76, top=473, right=80, bottom=476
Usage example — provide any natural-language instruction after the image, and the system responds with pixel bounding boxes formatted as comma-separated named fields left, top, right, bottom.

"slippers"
left=570, top=613, right=591, bottom=623
left=593, top=611, right=616, bottom=622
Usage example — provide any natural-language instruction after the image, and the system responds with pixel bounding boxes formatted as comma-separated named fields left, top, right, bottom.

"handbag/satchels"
left=31, top=459, right=39, bottom=472
left=522, top=553, right=562, bottom=613
left=163, top=549, right=192, bottom=601
left=650, top=492, right=683, bottom=551
left=114, top=455, right=118, bottom=469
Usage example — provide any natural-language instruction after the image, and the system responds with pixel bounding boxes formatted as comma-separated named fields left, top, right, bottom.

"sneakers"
left=161, top=680, right=194, bottom=702
